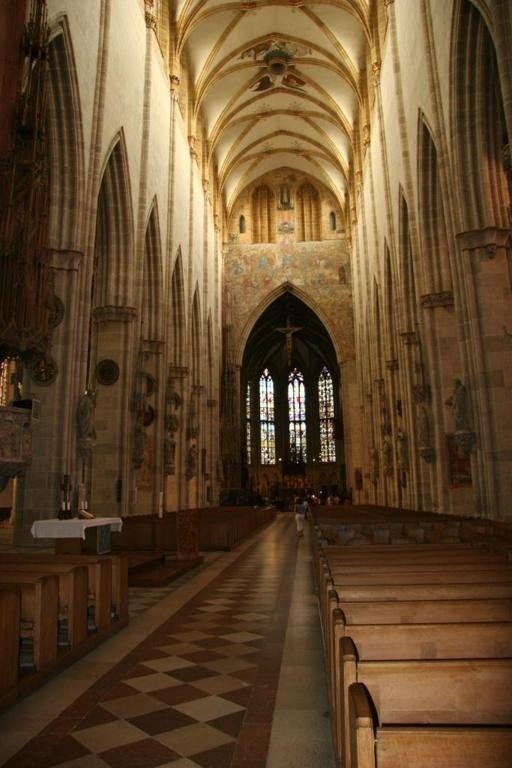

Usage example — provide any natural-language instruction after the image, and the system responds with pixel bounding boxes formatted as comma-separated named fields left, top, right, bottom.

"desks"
left=31, top=518, right=122, bottom=557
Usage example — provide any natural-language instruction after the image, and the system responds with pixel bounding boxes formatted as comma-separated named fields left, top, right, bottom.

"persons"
left=452, top=378, right=467, bottom=430
left=79, top=387, right=97, bottom=439
left=294, top=497, right=305, bottom=536
left=302, top=501, right=310, bottom=520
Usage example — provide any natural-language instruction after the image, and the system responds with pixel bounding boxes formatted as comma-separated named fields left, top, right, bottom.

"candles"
left=62, top=502, right=65, bottom=510
left=81, top=501, right=84, bottom=510
left=85, top=501, right=87, bottom=509
left=67, top=502, right=71, bottom=510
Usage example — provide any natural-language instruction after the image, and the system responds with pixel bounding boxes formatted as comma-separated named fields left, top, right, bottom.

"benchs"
left=306, top=503, right=511, bottom=547
left=0, top=551, right=112, bottom=627
left=111, top=555, right=128, bottom=618
left=0, top=562, right=88, bottom=648
left=109, top=502, right=276, bottom=549
left=0, top=573, right=59, bottom=663
left=0, top=581, right=20, bottom=698
